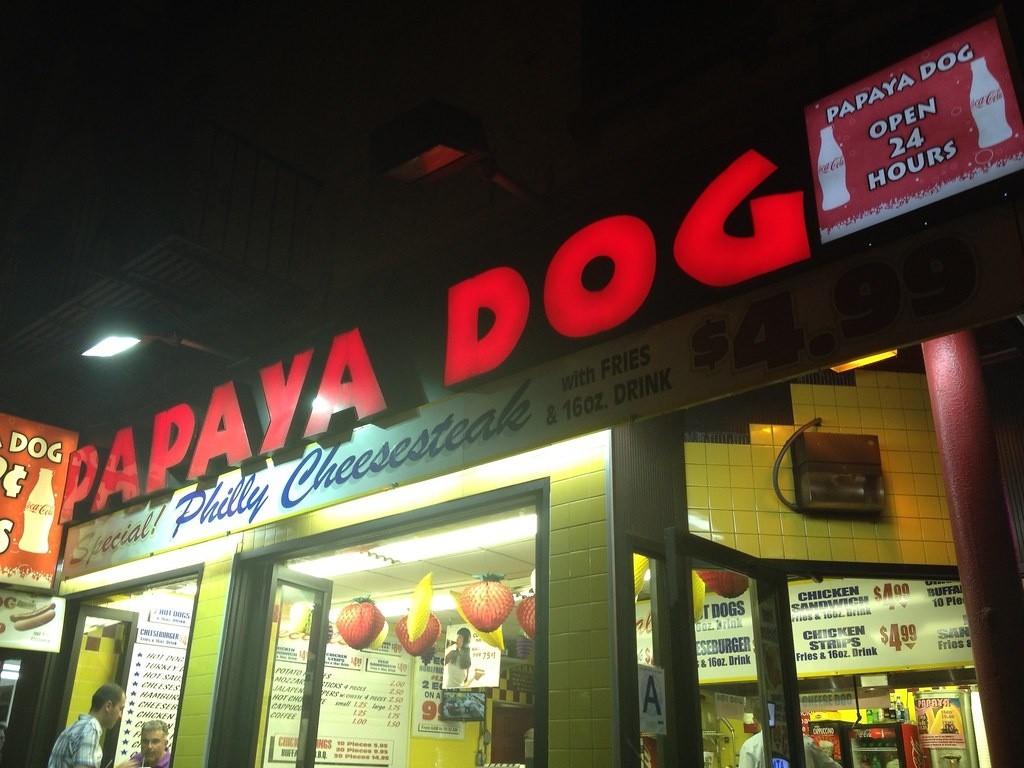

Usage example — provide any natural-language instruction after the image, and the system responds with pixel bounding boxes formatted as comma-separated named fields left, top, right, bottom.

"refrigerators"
left=848, top=724, right=923, bottom=768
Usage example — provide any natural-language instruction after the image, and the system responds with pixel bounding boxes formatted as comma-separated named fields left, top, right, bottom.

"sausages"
left=11, top=603, right=56, bottom=622
left=13, top=611, right=55, bottom=630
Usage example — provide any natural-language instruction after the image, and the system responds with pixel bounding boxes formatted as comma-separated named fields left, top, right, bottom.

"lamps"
left=81, top=314, right=238, bottom=359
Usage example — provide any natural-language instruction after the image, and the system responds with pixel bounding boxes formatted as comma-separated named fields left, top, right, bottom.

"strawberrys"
left=396, top=612, right=441, bottom=656
left=336, top=597, right=384, bottom=649
left=697, top=571, right=748, bottom=597
left=460, top=572, right=514, bottom=632
left=516, top=595, right=536, bottom=637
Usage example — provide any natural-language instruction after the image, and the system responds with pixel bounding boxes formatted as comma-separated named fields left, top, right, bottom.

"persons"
left=0, top=724, right=7, bottom=752
left=47, top=682, right=138, bottom=768
left=444, top=698, right=474, bottom=713
left=444, top=626, right=484, bottom=687
left=738, top=700, right=841, bottom=767
left=130, top=719, right=170, bottom=768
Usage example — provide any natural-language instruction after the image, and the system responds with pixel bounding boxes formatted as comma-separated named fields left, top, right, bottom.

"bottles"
left=969, top=57, right=1012, bottom=148
left=866, top=693, right=909, bottom=724
left=817, top=126, right=850, bottom=211
left=18, top=468, right=54, bottom=554
left=858, top=737, right=898, bottom=768
